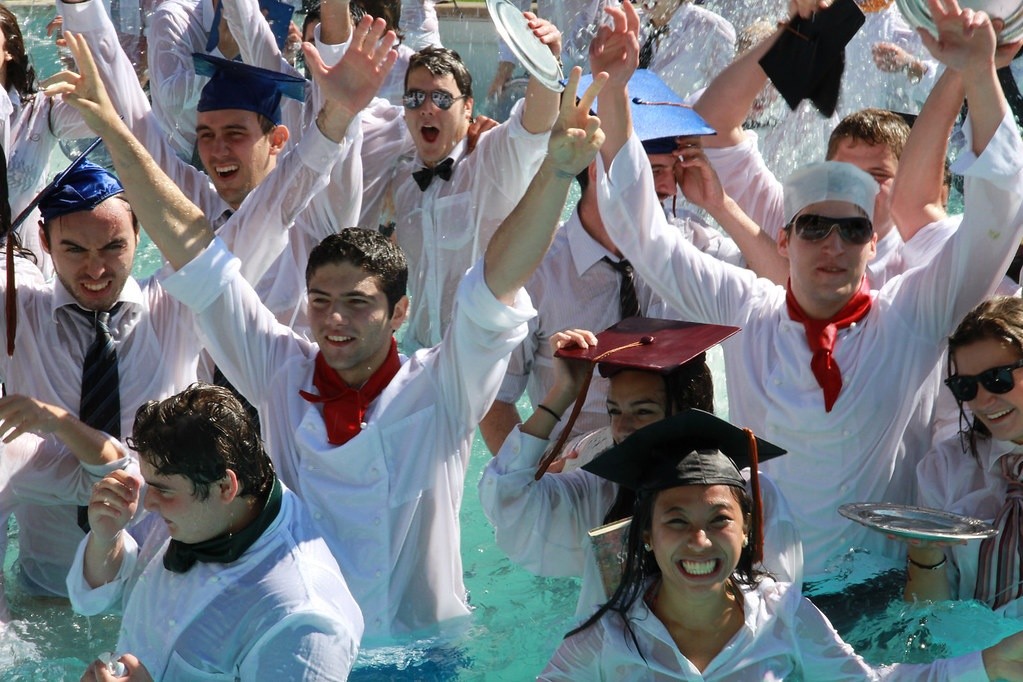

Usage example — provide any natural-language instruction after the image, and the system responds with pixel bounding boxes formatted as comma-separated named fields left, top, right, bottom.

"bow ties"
left=413, top=158, right=455, bottom=192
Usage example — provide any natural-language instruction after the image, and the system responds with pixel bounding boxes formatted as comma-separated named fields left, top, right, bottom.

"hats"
left=3, top=114, right=124, bottom=356
left=759, top=0, right=867, bottom=120
left=534, top=317, right=741, bottom=481
left=558, top=69, right=717, bottom=154
left=581, top=408, right=788, bottom=565
left=781, top=162, right=880, bottom=231
left=191, top=51, right=307, bottom=127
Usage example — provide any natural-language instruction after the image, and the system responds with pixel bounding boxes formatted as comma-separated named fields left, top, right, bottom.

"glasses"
left=784, top=214, right=875, bottom=245
left=402, top=88, right=467, bottom=111
left=944, top=360, right=1023, bottom=402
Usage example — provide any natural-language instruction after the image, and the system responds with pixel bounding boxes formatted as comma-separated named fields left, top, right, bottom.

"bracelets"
left=907, top=554, right=948, bottom=570
left=538, top=405, right=561, bottom=421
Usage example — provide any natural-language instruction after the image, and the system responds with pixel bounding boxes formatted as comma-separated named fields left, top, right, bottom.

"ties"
left=163, top=472, right=282, bottom=573
left=299, top=336, right=400, bottom=444
left=972, top=453, right=1023, bottom=607
left=68, top=302, right=122, bottom=533
left=601, top=255, right=641, bottom=321
left=635, top=21, right=670, bottom=69
left=787, top=274, right=873, bottom=414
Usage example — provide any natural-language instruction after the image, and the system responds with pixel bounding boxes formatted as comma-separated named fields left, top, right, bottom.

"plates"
left=484, top=0, right=565, bottom=93
left=838, top=502, right=999, bottom=543
left=895, top=0, right=1023, bottom=46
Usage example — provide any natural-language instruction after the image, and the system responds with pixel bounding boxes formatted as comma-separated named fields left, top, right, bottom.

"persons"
left=0, top=0, right=1023, bottom=682
